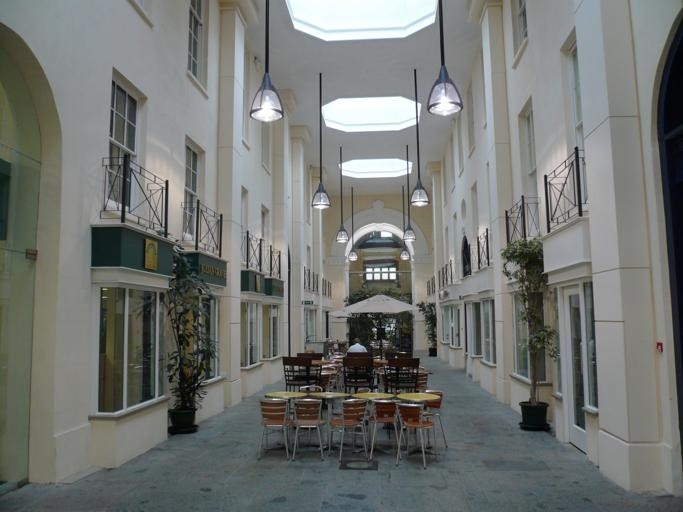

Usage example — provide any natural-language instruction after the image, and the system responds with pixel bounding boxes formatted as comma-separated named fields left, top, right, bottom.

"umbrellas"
left=327, top=307, right=374, bottom=336
left=344, top=292, right=424, bottom=359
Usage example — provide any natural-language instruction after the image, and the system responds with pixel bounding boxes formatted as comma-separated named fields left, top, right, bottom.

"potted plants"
left=500, top=239, right=560, bottom=432
left=163, top=246, right=218, bottom=434
left=416, top=300, right=438, bottom=356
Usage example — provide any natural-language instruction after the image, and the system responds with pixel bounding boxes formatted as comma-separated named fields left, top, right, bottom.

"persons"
left=346, top=337, right=367, bottom=357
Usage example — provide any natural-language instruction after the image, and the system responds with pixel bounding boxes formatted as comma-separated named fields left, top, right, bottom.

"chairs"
left=258, top=349, right=448, bottom=470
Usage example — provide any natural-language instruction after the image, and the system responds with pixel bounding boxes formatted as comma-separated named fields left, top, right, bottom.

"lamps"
left=399, top=69, right=429, bottom=261
left=310, top=73, right=357, bottom=261
left=426, top=1, right=463, bottom=116
left=249, top=1, right=283, bottom=122
